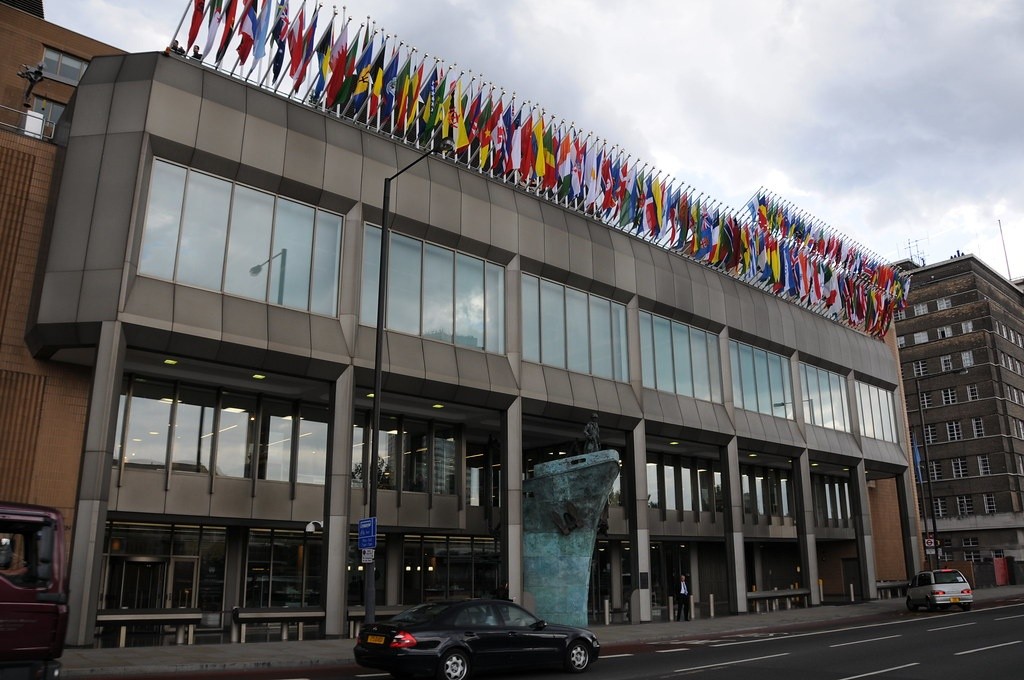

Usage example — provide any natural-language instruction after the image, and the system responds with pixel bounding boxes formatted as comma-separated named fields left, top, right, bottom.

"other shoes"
left=685, top=620, right=690, bottom=621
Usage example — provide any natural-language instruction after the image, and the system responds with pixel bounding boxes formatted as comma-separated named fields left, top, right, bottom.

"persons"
left=193, top=46, right=202, bottom=60
left=0, top=541, right=29, bottom=582
left=172, top=40, right=186, bottom=55
left=676, top=575, right=690, bottom=621
left=583, top=413, right=601, bottom=453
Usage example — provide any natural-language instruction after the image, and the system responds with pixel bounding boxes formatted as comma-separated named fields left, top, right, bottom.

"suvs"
left=905, top=568, right=973, bottom=613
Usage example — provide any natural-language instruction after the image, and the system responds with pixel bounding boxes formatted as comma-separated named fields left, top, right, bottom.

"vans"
left=0, top=502, right=71, bottom=680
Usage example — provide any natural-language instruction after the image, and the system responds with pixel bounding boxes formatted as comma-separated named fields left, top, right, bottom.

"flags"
left=188, top=0, right=913, bottom=338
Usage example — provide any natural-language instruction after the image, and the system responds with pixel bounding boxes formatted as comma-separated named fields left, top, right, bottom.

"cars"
left=352, top=597, right=602, bottom=680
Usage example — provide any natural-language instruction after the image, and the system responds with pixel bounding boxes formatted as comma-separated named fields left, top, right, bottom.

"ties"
left=683, top=583, right=687, bottom=596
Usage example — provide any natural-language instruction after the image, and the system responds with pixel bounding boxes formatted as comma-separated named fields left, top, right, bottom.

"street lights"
left=914, top=368, right=969, bottom=567
left=364, top=137, right=456, bottom=627
left=774, top=398, right=816, bottom=426
left=250, top=250, right=290, bottom=309
